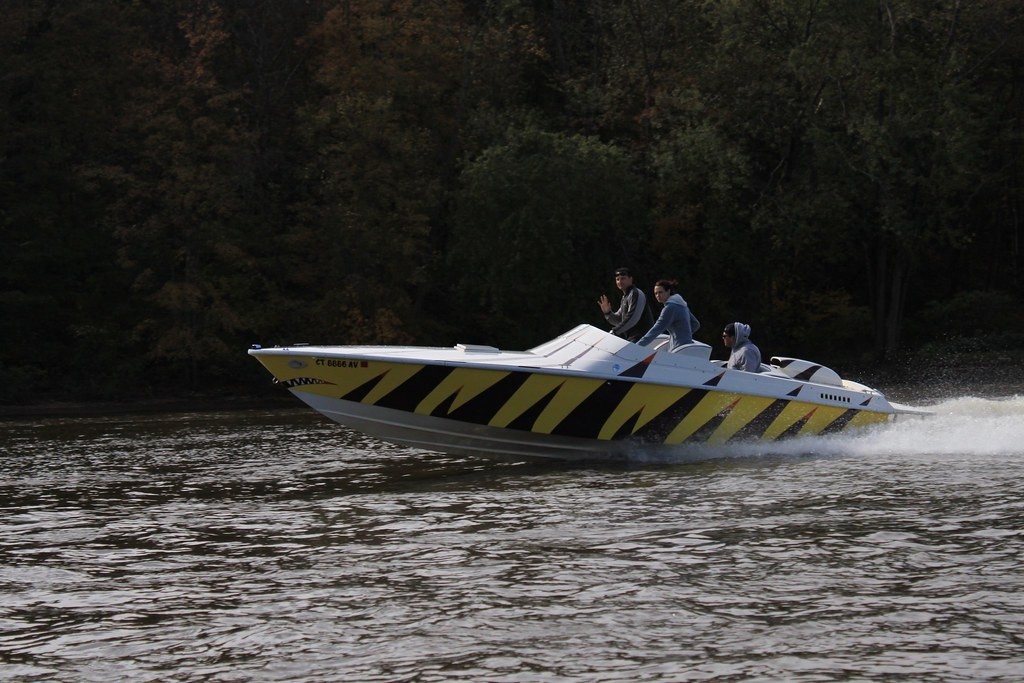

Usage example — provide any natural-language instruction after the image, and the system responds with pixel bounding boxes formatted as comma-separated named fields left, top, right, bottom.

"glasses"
left=722, top=334, right=731, bottom=340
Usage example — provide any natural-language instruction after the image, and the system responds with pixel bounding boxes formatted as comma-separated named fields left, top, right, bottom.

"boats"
left=248, top=315, right=932, bottom=467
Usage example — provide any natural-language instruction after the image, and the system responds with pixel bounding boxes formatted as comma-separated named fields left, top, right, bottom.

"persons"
left=635, top=280, right=701, bottom=354
left=721, top=322, right=761, bottom=373
left=597, top=267, right=654, bottom=341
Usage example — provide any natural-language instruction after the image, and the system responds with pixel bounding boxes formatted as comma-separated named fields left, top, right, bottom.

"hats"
left=724, top=322, right=736, bottom=337
left=614, top=268, right=632, bottom=278
